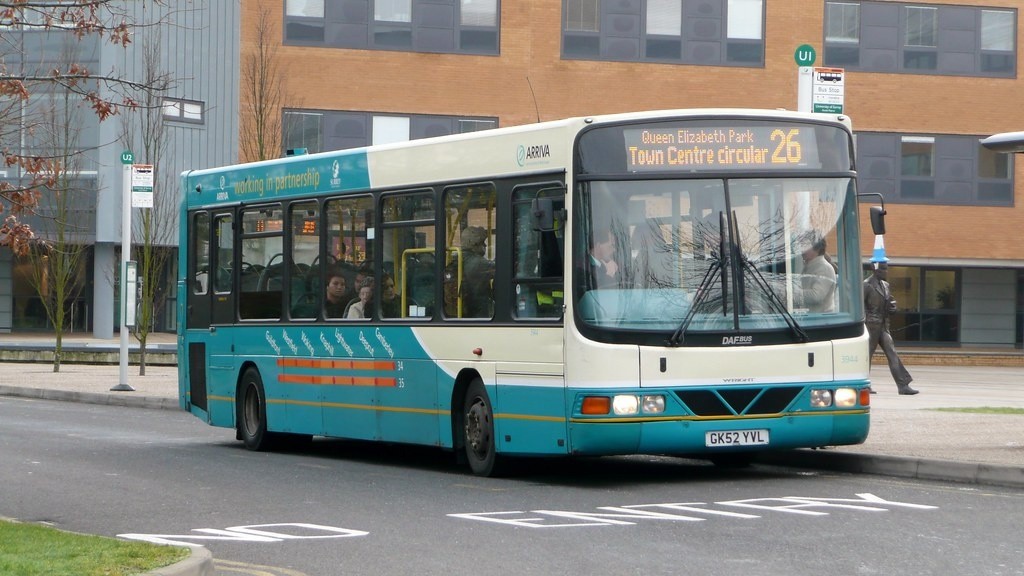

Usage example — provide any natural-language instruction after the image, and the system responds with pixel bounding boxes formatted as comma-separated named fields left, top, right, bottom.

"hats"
left=461, top=227, right=487, bottom=247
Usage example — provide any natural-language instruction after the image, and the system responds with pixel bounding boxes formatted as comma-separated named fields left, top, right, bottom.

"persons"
left=323, top=219, right=498, bottom=321
left=861, top=235, right=920, bottom=395
left=585, top=220, right=842, bottom=318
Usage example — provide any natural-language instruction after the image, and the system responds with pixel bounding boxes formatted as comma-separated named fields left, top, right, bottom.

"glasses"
left=384, top=284, right=393, bottom=290
left=332, top=281, right=344, bottom=286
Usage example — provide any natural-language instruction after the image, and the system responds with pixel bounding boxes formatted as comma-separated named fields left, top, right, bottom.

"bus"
left=176, top=104, right=888, bottom=477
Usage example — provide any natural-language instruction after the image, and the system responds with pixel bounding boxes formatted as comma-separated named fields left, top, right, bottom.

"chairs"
left=230, top=251, right=477, bottom=319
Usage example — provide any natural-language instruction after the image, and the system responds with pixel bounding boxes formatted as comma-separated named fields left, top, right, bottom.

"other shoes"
left=870, top=384, right=918, bottom=394
